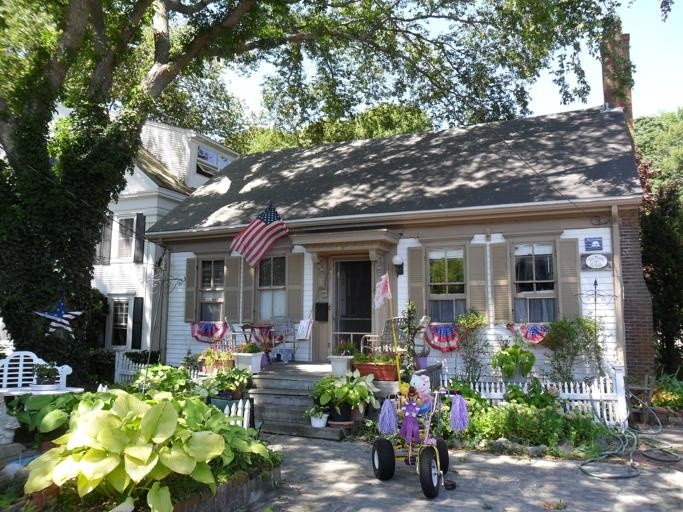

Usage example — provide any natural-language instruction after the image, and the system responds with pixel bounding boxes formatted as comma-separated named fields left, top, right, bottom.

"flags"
left=373, top=272, right=392, bottom=309
left=228, top=204, right=292, bottom=270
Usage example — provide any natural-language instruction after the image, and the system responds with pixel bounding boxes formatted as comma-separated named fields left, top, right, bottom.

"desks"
left=0, top=386, right=85, bottom=465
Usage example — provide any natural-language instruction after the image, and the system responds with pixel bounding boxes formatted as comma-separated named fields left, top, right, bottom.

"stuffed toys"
left=400, top=373, right=434, bottom=442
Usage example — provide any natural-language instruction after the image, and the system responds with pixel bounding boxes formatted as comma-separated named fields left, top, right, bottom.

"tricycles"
left=371, top=386, right=462, bottom=501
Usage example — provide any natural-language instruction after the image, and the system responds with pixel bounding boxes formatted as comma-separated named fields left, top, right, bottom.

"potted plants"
left=308, top=367, right=381, bottom=422
left=230, top=342, right=266, bottom=375
left=28, top=361, right=60, bottom=391
left=215, top=367, right=252, bottom=400
left=304, top=405, right=330, bottom=428
left=327, top=342, right=355, bottom=379
left=351, top=353, right=398, bottom=381
